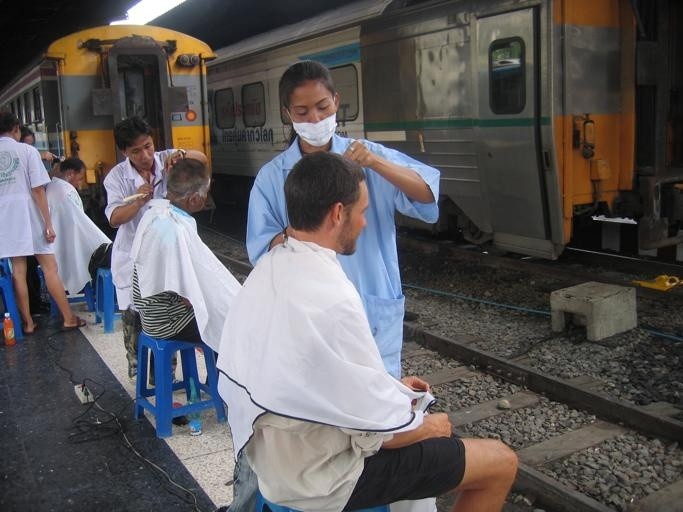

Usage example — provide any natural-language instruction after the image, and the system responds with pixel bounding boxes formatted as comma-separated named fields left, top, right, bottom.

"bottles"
left=187, top=392, right=205, bottom=436
left=3, top=312, right=16, bottom=347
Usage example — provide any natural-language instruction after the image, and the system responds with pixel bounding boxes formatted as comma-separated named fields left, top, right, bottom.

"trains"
left=0, top=25, right=219, bottom=214
left=205, top=0, right=683, bottom=261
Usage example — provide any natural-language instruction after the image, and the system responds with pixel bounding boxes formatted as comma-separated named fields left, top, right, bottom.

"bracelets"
left=176, top=148, right=187, bottom=159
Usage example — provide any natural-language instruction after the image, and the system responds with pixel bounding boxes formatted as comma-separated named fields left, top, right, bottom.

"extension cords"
left=74, top=383, right=94, bottom=404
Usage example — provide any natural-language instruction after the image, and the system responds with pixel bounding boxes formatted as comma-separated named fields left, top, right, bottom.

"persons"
left=214, top=150, right=518, bottom=512
left=0, top=110, right=88, bottom=335
left=244, top=60, right=441, bottom=386
left=127, top=150, right=237, bottom=403
left=46, top=155, right=65, bottom=181
left=38, top=157, right=115, bottom=308
left=101, top=116, right=208, bottom=394
left=17, top=126, right=57, bottom=316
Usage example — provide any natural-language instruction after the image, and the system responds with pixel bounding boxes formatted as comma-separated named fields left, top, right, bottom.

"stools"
left=135, top=333, right=227, bottom=437
left=256, top=489, right=390, bottom=512
left=0, top=257, right=126, bottom=340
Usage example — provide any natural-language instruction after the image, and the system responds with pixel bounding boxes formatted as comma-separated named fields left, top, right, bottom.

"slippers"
left=59, top=316, right=86, bottom=332
left=22, top=320, right=39, bottom=335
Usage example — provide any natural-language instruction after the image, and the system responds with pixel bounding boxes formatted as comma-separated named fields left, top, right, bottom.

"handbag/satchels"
left=88, top=243, right=113, bottom=279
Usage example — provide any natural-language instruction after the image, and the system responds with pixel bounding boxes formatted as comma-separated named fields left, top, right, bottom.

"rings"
left=348, top=147, right=354, bottom=153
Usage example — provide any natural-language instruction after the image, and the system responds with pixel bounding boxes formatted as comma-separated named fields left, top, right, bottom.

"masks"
left=289, top=112, right=338, bottom=147
